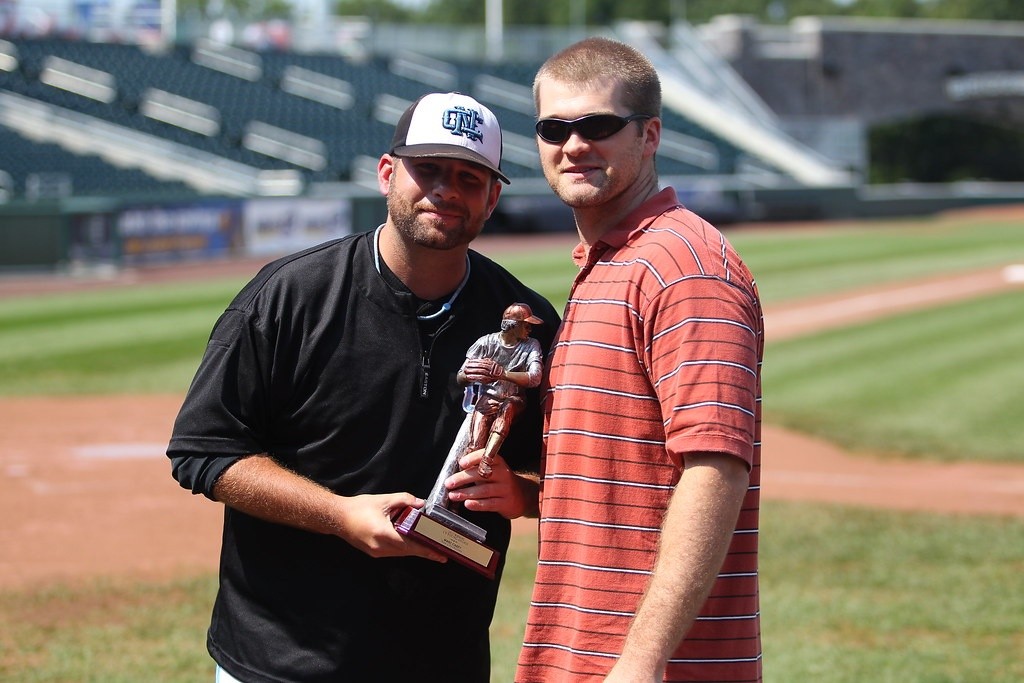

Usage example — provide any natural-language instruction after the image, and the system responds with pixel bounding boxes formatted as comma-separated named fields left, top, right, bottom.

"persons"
left=164, top=87, right=562, bottom=681
left=454, top=301, right=546, bottom=479
left=513, top=39, right=764, bottom=683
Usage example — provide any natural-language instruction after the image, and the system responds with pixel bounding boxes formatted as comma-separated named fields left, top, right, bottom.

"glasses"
left=534, top=113, right=650, bottom=143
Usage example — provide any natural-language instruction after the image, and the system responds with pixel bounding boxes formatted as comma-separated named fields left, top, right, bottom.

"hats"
left=388, top=90, right=512, bottom=187
